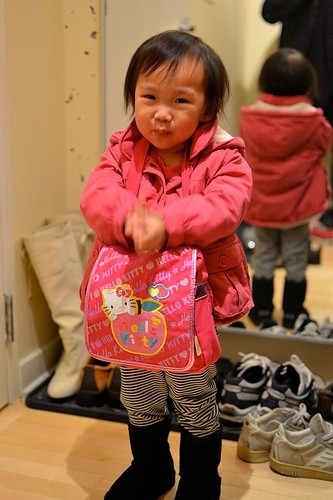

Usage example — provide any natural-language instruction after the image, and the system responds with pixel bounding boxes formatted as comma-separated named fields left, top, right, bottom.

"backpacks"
left=84, top=245, right=222, bottom=374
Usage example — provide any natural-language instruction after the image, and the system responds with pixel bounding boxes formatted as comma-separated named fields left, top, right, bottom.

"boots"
left=174, top=427, right=222, bottom=500
left=282, top=275, right=309, bottom=328
left=103, top=417, right=175, bottom=500
left=22, top=211, right=95, bottom=401
left=247, top=274, right=274, bottom=326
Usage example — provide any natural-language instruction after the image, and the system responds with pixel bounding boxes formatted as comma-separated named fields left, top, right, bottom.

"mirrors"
left=101, top=0, right=333, bottom=346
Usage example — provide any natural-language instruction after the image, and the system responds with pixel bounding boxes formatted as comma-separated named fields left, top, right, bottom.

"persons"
left=234, top=45, right=332, bottom=330
left=261, top=0, right=333, bottom=125
left=78, top=28, right=255, bottom=500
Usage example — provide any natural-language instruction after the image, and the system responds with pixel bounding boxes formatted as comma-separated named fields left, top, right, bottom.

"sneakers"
left=76, top=355, right=124, bottom=409
left=236, top=403, right=332, bottom=480
left=217, top=351, right=325, bottom=424
left=260, top=311, right=333, bottom=340
